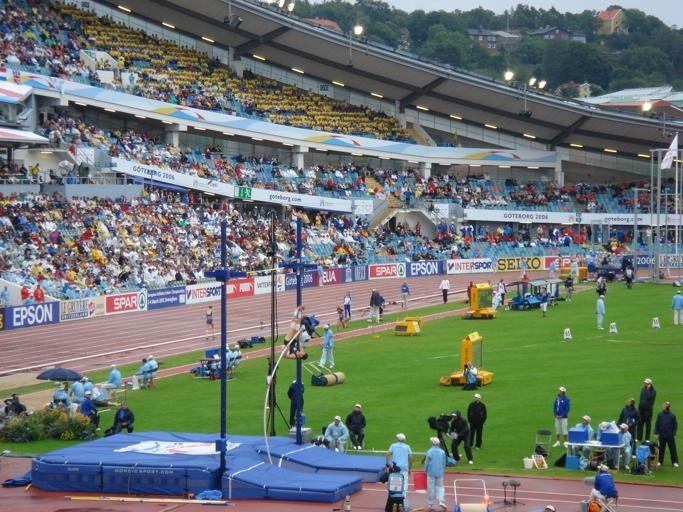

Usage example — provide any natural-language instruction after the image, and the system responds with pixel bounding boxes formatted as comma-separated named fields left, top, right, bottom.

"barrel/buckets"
left=415, top=473, right=428, bottom=492
left=523, top=457, right=535, bottom=470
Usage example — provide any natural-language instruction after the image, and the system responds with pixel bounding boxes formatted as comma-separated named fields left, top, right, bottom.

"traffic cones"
left=481, top=491, right=492, bottom=504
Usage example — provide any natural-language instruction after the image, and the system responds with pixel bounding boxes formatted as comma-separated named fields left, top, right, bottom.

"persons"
left=1, top=159, right=274, bottom=306
left=384, top=393, right=487, bottom=510
left=670, top=290, right=683, bottom=325
left=440, top=276, right=451, bottom=303
left=37, top=112, right=678, bottom=216
left=53, top=356, right=159, bottom=437
left=288, top=380, right=305, bottom=427
left=550, top=377, right=678, bottom=506
left=301, top=288, right=386, bottom=368
left=205, top=304, right=216, bottom=340
left=323, top=403, right=366, bottom=454
left=1, top=0, right=407, bottom=142
left=464, top=361, right=477, bottom=390
left=270, top=206, right=682, bottom=269
left=496, top=272, right=576, bottom=318
left=400, top=281, right=411, bottom=309
left=595, top=266, right=634, bottom=330
left=190, top=341, right=242, bottom=382
left=283, top=305, right=309, bottom=360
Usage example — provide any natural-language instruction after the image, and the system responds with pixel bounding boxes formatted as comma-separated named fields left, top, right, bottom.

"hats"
left=396, top=433, right=406, bottom=441
left=334, top=416, right=341, bottom=422
left=429, top=436, right=440, bottom=445
left=355, top=403, right=361, bottom=409
left=581, top=415, right=591, bottom=423
left=473, top=393, right=481, bottom=400
left=599, top=294, right=605, bottom=298
left=559, top=386, right=567, bottom=393
left=84, top=390, right=91, bottom=395
left=322, top=325, right=329, bottom=330
left=597, top=463, right=609, bottom=472
left=544, top=505, right=556, bottom=512
left=643, top=378, right=653, bottom=386
left=619, top=423, right=629, bottom=432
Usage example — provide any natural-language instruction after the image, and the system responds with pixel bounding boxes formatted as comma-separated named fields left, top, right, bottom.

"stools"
left=599, top=498, right=616, bottom=512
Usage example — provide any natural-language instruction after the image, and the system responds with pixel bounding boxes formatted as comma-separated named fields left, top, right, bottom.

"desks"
left=565, top=442, right=625, bottom=472
left=198, top=358, right=220, bottom=376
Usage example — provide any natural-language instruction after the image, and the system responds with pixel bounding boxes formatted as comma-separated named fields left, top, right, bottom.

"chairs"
left=209, top=355, right=242, bottom=379
left=631, top=446, right=659, bottom=471
left=534, top=430, right=552, bottom=458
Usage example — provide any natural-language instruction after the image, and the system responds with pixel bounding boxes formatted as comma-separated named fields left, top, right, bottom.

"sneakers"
left=654, top=461, right=661, bottom=467
left=550, top=440, right=560, bottom=448
left=353, top=446, right=362, bottom=451
left=671, top=462, right=679, bottom=467
left=467, top=459, right=474, bottom=465
left=438, top=502, right=447, bottom=509
left=623, top=464, right=629, bottom=471
left=562, top=441, right=568, bottom=449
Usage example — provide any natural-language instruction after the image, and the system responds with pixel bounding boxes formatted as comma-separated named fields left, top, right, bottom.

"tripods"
left=494, top=486, right=526, bottom=506
